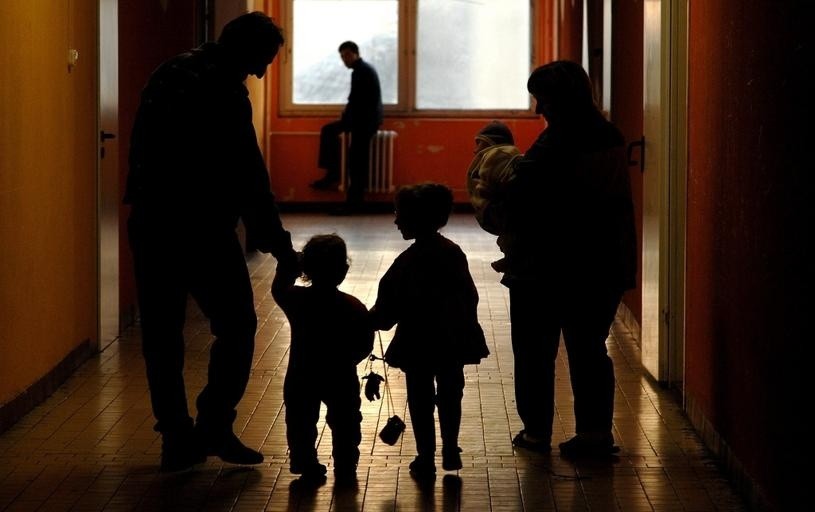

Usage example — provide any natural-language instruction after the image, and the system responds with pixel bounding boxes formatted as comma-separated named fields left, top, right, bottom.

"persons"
left=369, top=183, right=489, bottom=474
left=272, top=234, right=374, bottom=477
left=470, top=61, right=636, bottom=459
left=308, top=41, right=382, bottom=192
left=469, top=120, right=524, bottom=273
left=122, top=11, right=295, bottom=467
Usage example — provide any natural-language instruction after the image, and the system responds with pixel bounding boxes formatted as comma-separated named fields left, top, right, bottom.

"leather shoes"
left=159, top=425, right=263, bottom=470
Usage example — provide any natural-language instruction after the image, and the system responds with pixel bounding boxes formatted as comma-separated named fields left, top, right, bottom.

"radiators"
left=338, top=129, right=399, bottom=194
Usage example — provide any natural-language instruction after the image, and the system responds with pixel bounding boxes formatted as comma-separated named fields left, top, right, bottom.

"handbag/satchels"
left=379, top=415, right=405, bottom=448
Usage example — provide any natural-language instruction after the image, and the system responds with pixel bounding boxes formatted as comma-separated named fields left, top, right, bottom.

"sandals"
left=509, top=431, right=553, bottom=453
left=560, top=433, right=621, bottom=452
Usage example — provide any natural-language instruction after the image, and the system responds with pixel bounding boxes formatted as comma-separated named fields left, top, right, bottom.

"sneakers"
left=442, top=455, right=463, bottom=472
left=408, top=457, right=437, bottom=471
left=332, top=462, right=357, bottom=478
left=290, top=454, right=326, bottom=476
